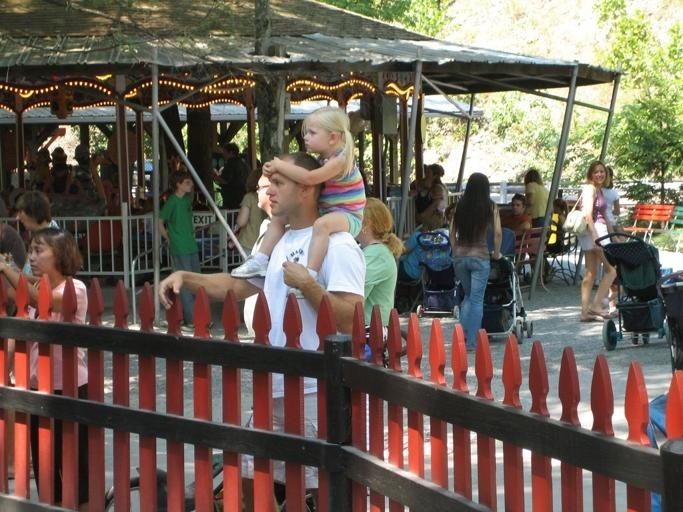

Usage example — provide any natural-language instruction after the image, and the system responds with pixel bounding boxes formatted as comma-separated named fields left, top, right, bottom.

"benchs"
left=623, top=202, right=675, bottom=241
left=662, top=207, right=682, bottom=251
left=509, top=227, right=556, bottom=292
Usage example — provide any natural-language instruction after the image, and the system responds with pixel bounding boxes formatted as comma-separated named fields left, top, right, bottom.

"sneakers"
left=229, top=258, right=267, bottom=277
left=286, top=281, right=325, bottom=298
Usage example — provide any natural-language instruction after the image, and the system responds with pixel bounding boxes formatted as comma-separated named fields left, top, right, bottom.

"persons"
left=408, top=164, right=568, bottom=350
left=156, top=107, right=367, bottom=336
left=2, top=145, right=119, bottom=512
left=575, top=161, right=620, bottom=321
left=355, top=198, right=404, bottom=362
left=155, top=152, right=369, bottom=511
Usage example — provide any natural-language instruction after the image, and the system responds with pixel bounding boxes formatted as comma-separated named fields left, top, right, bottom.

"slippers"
left=580, top=306, right=617, bottom=322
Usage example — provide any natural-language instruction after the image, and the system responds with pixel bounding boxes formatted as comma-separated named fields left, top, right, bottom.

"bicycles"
left=103, top=406, right=317, bottom=511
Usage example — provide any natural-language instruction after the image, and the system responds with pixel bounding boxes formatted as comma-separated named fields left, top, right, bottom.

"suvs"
left=130, top=159, right=154, bottom=186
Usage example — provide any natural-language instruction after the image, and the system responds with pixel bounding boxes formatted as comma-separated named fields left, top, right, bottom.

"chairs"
left=549, top=230, right=582, bottom=287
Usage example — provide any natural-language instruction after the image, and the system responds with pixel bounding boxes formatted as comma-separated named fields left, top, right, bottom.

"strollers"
left=453, top=227, right=533, bottom=344
left=416, top=231, right=464, bottom=319
left=594, top=232, right=671, bottom=351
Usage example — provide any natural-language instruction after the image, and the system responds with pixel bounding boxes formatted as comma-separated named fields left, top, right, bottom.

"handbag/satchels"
left=561, top=211, right=587, bottom=234
